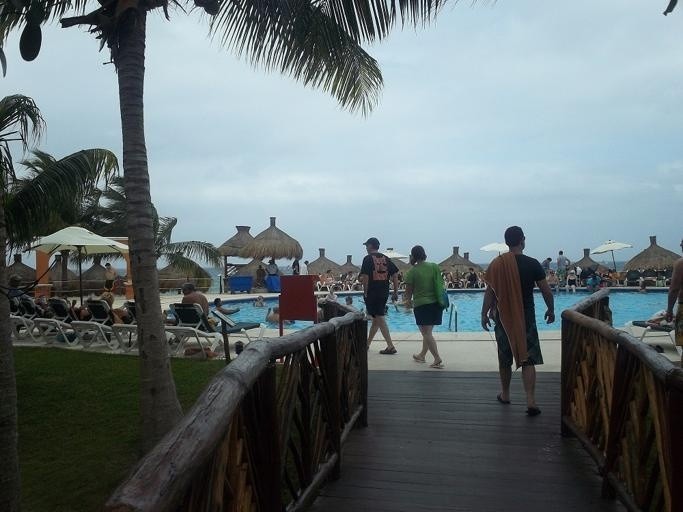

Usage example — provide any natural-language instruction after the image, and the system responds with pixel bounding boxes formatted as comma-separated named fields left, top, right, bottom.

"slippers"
left=526, top=408, right=541, bottom=415
left=497, top=393, right=510, bottom=404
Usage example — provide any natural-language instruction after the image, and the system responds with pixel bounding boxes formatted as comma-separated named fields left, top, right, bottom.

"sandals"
left=412, top=354, right=424, bottom=363
left=428, top=361, right=443, bottom=369
left=380, top=347, right=396, bottom=354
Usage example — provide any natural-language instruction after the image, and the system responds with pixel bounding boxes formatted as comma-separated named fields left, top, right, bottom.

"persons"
left=481, top=226, right=553, bottom=416
left=666, top=240, right=683, bottom=368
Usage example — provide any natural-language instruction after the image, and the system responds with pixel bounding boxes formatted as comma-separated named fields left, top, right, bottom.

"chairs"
left=8, top=291, right=268, bottom=360
left=624, top=269, right=670, bottom=287
left=632, top=318, right=675, bottom=350
left=316, top=273, right=364, bottom=292
left=440, top=271, right=487, bottom=289
left=601, top=273, right=620, bottom=287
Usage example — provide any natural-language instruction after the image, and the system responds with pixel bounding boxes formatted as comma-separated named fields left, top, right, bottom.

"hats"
left=362, top=237, right=379, bottom=247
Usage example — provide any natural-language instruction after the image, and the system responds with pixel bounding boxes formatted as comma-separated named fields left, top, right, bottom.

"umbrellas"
left=26, top=227, right=129, bottom=306
left=590, top=240, right=633, bottom=271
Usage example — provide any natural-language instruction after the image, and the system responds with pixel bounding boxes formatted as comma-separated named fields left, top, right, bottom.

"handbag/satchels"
left=431, top=263, right=448, bottom=308
